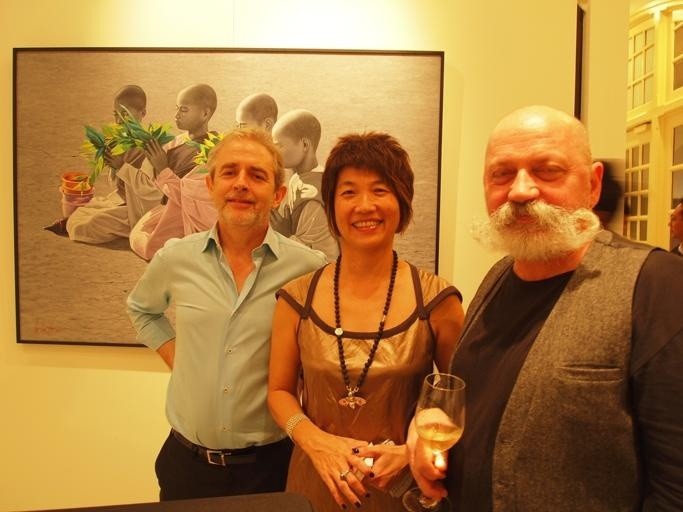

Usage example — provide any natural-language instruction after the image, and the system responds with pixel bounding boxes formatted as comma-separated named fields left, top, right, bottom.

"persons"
left=409, top=104, right=682, bottom=512
left=237, top=94, right=277, bottom=142
left=265, top=134, right=469, bottom=509
left=126, top=132, right=329, bottom=503
left=665, top=203, right=682, bottom=256
left=128, top=85, right=215, bottom=259
left=64, top=85, right=149, bottom=244
left=265, top=111, right=344, bottom=265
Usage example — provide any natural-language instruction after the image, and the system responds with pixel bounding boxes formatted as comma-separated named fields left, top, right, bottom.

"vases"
left=58, top=172, right=93, bottom=220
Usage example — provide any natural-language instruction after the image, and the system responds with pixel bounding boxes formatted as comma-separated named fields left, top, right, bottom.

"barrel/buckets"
left=58, top=173, right=96, bottom=219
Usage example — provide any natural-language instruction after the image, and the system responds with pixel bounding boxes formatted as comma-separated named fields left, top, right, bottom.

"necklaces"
left=331, top=251, right=402, bottom=408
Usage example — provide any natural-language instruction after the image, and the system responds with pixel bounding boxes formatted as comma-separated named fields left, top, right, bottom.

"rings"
left=339, top=470, right=351, bottom=479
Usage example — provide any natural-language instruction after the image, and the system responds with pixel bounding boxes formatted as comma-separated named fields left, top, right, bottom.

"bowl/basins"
left=58, top=172, right=96, bottom=217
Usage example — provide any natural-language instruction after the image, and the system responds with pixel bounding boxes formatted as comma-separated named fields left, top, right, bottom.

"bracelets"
left=285, top=412, right=312, bottom=443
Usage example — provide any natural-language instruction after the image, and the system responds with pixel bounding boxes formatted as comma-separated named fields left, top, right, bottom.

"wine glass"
left=401, top=372, right=468, bottom=512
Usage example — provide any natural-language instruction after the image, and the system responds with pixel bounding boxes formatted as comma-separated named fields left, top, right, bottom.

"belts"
left=170, top=429, right=291, bottom=466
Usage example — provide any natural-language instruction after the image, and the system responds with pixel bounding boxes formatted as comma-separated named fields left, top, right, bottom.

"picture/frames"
left=10, top=45, right=446, bottom=349
